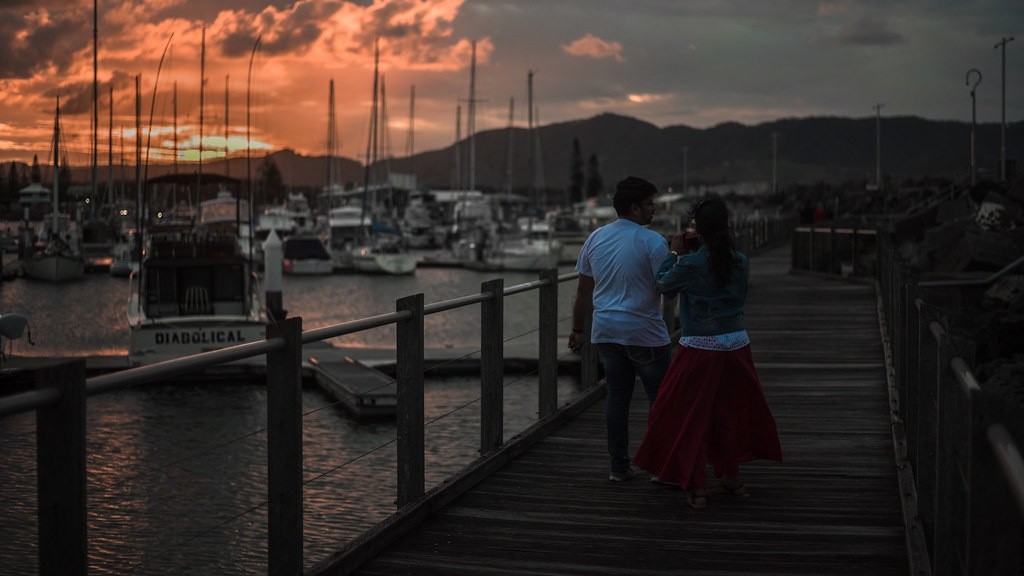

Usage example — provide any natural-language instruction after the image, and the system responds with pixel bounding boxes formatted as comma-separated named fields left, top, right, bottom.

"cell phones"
left=684, top=232, right=702, bottom=251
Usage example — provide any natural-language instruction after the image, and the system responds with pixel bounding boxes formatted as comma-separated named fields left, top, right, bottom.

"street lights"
left=994, top=36, right=1015, bottom=183
left=871, top=101, right=886, bottom=186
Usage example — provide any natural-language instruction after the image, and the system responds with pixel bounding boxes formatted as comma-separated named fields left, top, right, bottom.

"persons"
left=631, top=200, right=783, bottom=509
left=567, top=176, right=681, bottom=486
left=798, top=200, right=828, bottom=224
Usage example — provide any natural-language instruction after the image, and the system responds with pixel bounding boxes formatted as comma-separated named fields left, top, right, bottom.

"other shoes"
left=650, top=474, right=661, bottom=482
left=610, top=468, right=636, bottom=482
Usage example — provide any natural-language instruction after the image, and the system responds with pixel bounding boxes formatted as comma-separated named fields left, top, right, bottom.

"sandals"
left=689, top=490, right=708, bottom=509
left=708, top=481, right=750, bottom=500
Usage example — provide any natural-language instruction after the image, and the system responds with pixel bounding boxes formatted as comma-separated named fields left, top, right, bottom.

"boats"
left=124, top=215, right=265, bottom=364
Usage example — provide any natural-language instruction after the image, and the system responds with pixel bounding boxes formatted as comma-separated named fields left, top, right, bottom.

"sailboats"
left=0, top=0, right=694, bottom=283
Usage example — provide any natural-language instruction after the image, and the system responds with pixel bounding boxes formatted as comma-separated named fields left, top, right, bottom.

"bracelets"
left=573, top=327, right=584, bottom=333
left=670, top=250, right=680, bottom=255
